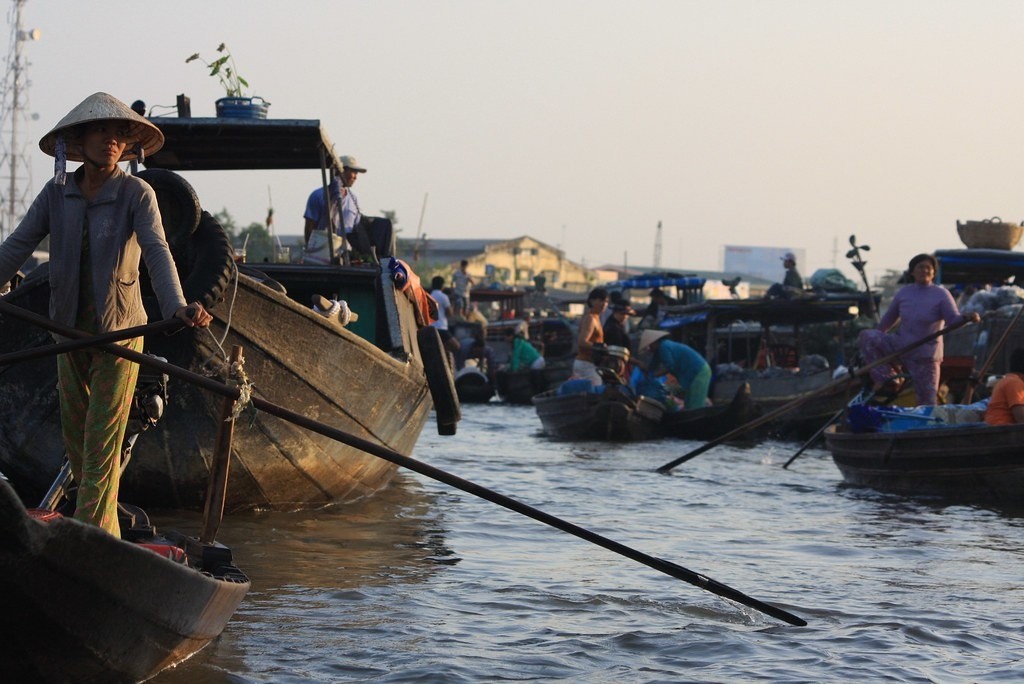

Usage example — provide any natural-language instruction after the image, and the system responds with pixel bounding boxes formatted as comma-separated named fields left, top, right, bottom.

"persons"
left=984, top=345, right=1024, bottom=425
left=429, top=259, right=712, bottom=411
left=781, top=253, right=804, bottom=289
left=0, top=92, right=214, bottom=540
left=857, top=254, right=980, bottom=408
left=304, top=157, right=371, bottom=263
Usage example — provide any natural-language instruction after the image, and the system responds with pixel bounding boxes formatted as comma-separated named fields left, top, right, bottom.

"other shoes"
left=880, top=376, right=905, bottom=397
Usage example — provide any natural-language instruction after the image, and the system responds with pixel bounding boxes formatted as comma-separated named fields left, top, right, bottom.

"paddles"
left=784, top=409, right=842, bottom=469
left=960, top=306, right=1024, bottom=404
left=0, top=304, right=207, bottom=365
left=0, top=298, right=810, bottom=627
left=658, top=315, right=972, bottom=474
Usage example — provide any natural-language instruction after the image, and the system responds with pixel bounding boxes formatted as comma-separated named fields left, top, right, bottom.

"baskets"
left=215, top=96, right=271, bottom=120
left=957, top=217, right=1024, bottom=249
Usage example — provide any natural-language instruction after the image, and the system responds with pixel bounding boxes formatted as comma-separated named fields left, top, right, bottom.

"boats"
left=0, top=119, right=435, bottom=517
left=656, top=382, right=770, bottom=440
left=0, top=471, right=253, bottom=684
left=531, top=389, right=668, bottom=442
left=824, top=422, right=1024, bottom=505
left=421, top=247, right=1023, bottom=438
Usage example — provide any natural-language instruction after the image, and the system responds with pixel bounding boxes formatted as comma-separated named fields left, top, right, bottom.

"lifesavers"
left=129, top=167, right=236, bottom=312
left=417, top=325, right=461, bottom=437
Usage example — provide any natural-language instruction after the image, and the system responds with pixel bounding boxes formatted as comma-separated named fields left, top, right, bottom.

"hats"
left=612, top=303, right=637, bottom=314
left=339, top=155, right=367, bottom=173
left=780, top=252, right=795, bottom=264
left=638, top=329, right=671, bottom=355
left=38, top=92, right=165, bottom=163
